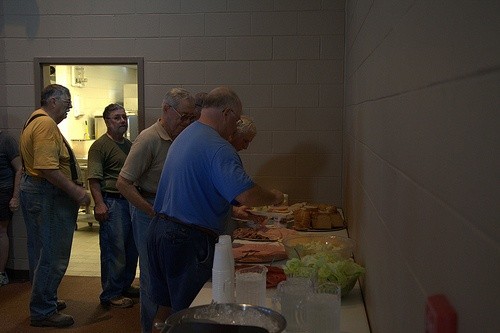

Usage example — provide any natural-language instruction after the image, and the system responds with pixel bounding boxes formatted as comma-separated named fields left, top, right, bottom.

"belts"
left=140, top=191, right=157, bottom=197
left=153, top=212, right=219, bottom=243
left=101, top=189, right=128, bottom=201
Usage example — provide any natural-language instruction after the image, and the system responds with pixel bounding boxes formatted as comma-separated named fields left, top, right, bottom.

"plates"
left=232, top=204, right=346, bottom=287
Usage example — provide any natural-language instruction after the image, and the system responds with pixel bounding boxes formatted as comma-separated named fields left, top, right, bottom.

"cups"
left=211, top=235, right=234, bottom=305
left=235, top=265, right=268, bottom=307
left=276, top=279, right=341, bottom=333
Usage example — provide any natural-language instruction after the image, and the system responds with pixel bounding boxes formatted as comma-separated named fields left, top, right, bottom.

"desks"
left=189, top=206, right=371, bottom=333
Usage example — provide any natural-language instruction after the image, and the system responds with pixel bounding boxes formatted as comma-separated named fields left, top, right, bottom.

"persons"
left=20, top=84, right=91, bottom=328
left=87, top=104, right=140, bottom=308
left=116, top=87, right=283, bottom=333
left=0, top=131, right=22, bottom=287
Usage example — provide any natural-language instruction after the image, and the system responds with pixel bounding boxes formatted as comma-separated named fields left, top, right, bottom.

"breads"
left=294, top=204, right=345, bottom=230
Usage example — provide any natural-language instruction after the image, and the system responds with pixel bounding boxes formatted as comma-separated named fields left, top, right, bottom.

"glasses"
left=108, top=116, right=128, bottom=120
left=166, top=103, right=197, bottom=124
left=222, top=105, right=243, bottom=128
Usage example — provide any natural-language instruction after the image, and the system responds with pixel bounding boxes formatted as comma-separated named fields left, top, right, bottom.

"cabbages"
left=283, top=239, right=366, bottom=295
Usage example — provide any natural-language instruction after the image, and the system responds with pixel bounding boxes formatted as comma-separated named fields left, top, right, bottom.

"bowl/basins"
left=282, top=235, right=353, bottom=260
left=285, top=253, right=360, bottom=299
left=160, top=303, right=287, bottom=332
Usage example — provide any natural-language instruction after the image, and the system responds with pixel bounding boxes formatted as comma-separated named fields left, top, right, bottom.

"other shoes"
left=57, top=301, right=68, bottom=310
left=110, top=296, right=133, bottom=307
left=0, top=272, right=9, bottom=285
left=29, top=312, right=75, bottom=327
left=124, top=288, right=141, bottom=298
